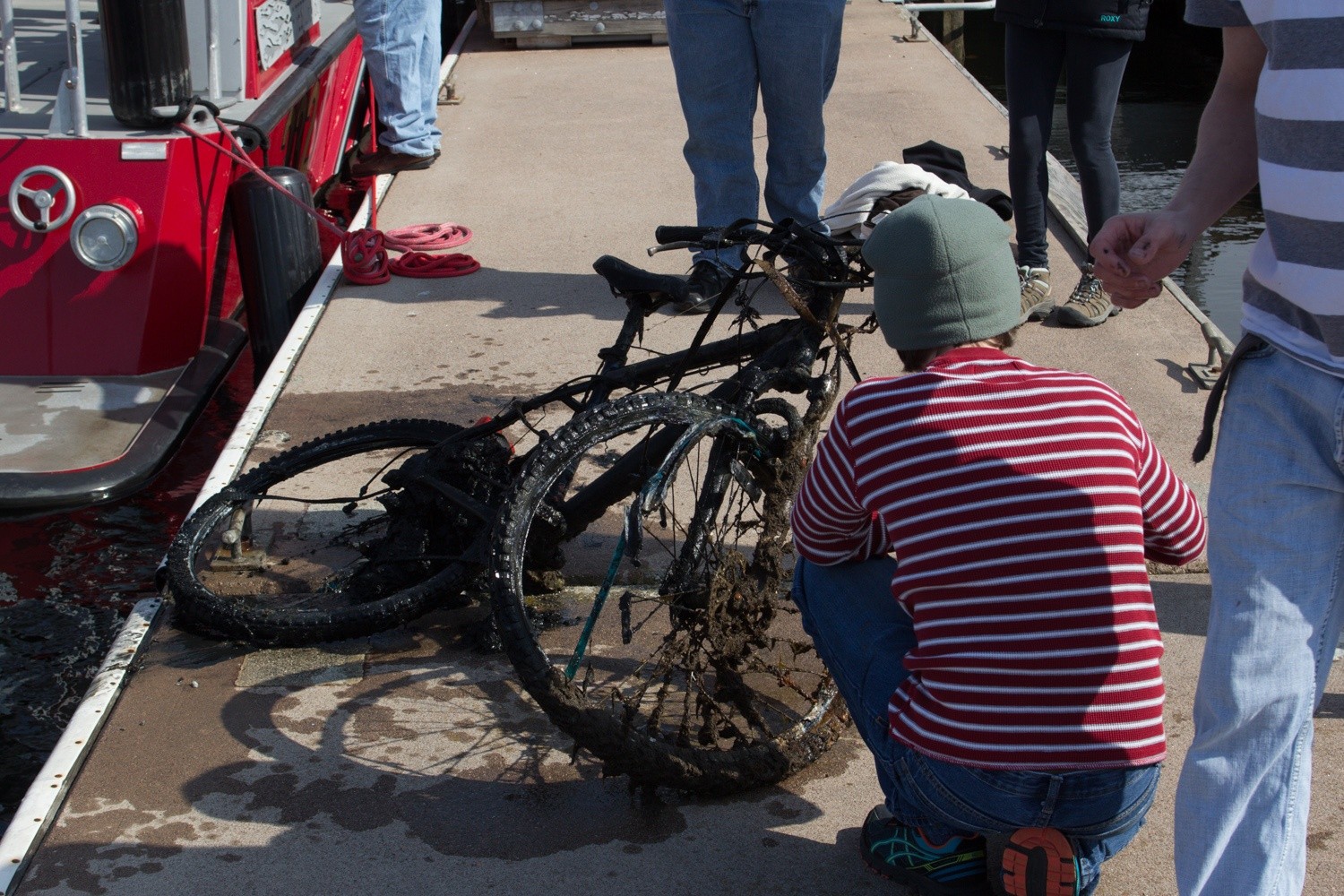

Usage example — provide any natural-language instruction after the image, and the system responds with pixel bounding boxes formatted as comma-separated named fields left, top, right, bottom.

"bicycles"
left=164, top=210, right=880, bottom=804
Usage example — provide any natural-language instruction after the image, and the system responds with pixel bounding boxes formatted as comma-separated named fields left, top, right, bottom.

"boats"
left=0, top=0, right=370, bottom=517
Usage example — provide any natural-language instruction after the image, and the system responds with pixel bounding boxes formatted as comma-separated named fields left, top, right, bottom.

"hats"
left=862, top=195, right=1020, bottom=350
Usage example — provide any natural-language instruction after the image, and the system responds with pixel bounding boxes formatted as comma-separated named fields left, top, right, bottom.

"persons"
left=345, top=0, right=445, bottom=178
left=792, top=193, right=1205, bottom=896
left=1080, top=0, right=1344, bottom=896
left=662, top=0, right=849, bottom=314
left=1003, top=0, right=1150, bottom=326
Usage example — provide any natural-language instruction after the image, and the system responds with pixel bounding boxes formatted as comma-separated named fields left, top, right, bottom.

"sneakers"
left=1000, top=826, right=1081, bottom=896
left=1011, top=265, right=1055, bottom=328
left=1059, top=260, right=1126, bottom=328
left=860, top=803, right=988, bottom=896
left=787, top=241, right=829, bottom=300
left=673, top=260, right=739, bottom=313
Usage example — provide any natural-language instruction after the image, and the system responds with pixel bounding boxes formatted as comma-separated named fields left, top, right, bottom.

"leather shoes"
left=350, top=144, right=441, bottom=176
